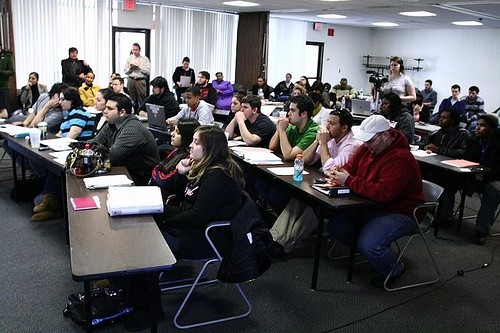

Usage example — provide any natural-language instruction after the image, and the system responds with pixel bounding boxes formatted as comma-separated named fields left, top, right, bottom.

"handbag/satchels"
left=62, top=281, right=137, bottom=333
left=65, top=141, right=112, bottom=178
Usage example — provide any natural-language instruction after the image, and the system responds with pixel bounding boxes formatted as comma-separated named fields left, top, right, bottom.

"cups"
left=37, top=122, right=48, bottom=139
left=29, top=129, right=41, bottom=148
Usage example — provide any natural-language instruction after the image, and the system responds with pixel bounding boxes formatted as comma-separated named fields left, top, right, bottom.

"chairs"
left=328, top=180, right=456, bottom=292
left=163, top=190, right=264, bottom=329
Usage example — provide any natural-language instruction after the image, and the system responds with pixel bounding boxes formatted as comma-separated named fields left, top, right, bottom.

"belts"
left=129, top=76, right=145, bottom=80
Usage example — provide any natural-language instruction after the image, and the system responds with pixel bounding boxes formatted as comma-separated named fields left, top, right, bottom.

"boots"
left=125, top=290, right=164, bottom=331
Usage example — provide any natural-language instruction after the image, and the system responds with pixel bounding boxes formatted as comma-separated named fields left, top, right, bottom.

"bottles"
left=360, top=88, right=363, bottom=99
left=82, top=144, right=95, bottom=175
left=293, top=154, right=304, bottom=181
left=342, top=96, right=346, bottom=109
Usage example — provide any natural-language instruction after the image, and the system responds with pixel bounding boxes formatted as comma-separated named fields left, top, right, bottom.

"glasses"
left=365, top=132, right=384, bottom=145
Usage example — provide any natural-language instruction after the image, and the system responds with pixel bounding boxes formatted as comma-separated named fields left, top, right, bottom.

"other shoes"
left=258, top=228, right=273, bottom=246
left=33, top=192, right=56, bottom=212
left=473, top=229, right=487, bottom=245
left=31, top=209, right=61, bottom=222
left=430, top=212, right=457, bottom=228
left=370, top=266, right=406, bottom=289
left=263, top=241, right=289, bottom=260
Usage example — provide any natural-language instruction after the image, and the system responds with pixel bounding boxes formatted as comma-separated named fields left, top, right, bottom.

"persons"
left=0, top=71, right=245, bottom=333
left=124, top=43, right=151, bottom=114
left=61, top=47, right=93, bottom=90
left=371, top=56, right=417, bottom=110
left=274, top=73, right=358, bottom=126
left=252, top=76, right=270, bottom=99
left=222, top=90, right=426, bottom=289
left=172, top=57, right=195, bottom=104
left=406, top=80, right=500, bottom=245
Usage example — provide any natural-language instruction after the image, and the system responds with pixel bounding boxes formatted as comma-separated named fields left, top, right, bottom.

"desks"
left=412, top=147, right=490, bottom=240
left=228, top=148, right=377, bottom=291
left=6, top=131, right=78, bottom=184
left=65, top=166, right=177, bottom=333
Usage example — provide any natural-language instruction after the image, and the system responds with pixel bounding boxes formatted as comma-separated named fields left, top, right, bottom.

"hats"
left=351, top=114, right=390, bottom=141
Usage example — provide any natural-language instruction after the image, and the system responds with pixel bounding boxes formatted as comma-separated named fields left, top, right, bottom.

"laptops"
left=145, top=102, right=174, bottom=132
left=351, top=99, right=373, bottom=116
